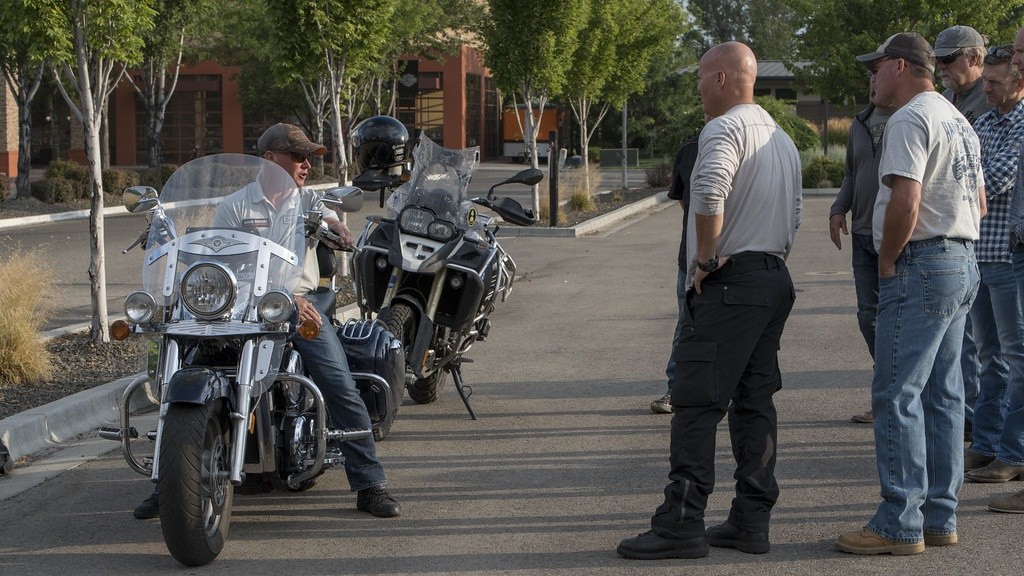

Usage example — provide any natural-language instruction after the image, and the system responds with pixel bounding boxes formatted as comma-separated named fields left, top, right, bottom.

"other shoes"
left=964, top=430, right=974, bottom=442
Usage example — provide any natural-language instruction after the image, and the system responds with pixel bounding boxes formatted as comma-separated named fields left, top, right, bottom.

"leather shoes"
left=923, top=533, right=958, bottom=544
left=964, top=458, right=1024, bottom=482
left=964, top=451, right=996, bottom=471
left=836, top=526, right=924, bottom=555
left=357, top=488, right=401, bottom=517
left=988, top=489, right=1024, bottom=512
left=133, top=484, right=159, bottom=520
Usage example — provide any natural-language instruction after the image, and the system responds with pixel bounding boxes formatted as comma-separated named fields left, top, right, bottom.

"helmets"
left=351, top=115, right=410, bottom=175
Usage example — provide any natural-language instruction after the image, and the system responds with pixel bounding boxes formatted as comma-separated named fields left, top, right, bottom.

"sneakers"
left=726, top=399, right=732, bottom=411
left=651, top=391, right=672, bottom=413
left=706, top=520, right=770, bottom=554
left=616, top=528, right=711, bottom=560
left=852, top=410, right=874, bottom=422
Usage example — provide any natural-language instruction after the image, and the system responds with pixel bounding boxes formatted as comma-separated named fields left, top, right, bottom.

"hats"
left=257, top=123, right=327, bottom=156
left=856, top=32, right=936, bottom=75
left=933, top=25, right=983, bottom=57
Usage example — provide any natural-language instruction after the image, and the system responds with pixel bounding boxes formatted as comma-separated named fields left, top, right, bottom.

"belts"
left=720, top=259, right=785, bottom=275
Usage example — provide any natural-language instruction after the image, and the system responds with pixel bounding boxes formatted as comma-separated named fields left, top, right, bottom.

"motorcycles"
left=98, top=154, right=406, bottom=567
left=344, top=132, right=545, bottom=442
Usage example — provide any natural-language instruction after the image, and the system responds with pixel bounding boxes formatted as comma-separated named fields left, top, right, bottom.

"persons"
left=614, top=40, right=804, bottom=559
left=829, top=18, right=1023, bottom=553
left=132, top=123, right=404, bottom=521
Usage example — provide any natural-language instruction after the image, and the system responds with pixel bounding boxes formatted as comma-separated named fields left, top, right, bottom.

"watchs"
left=695, top=254, right=720, bottom=273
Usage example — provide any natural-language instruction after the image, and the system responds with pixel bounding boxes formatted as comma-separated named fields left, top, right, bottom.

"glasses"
left=989, top=46, right=1012, bottom=58
left=870, top=57, right=907, bottom=74
left=272, top=150, right=312, bottom=163
left=936, top=52, right=967, bottom=64
